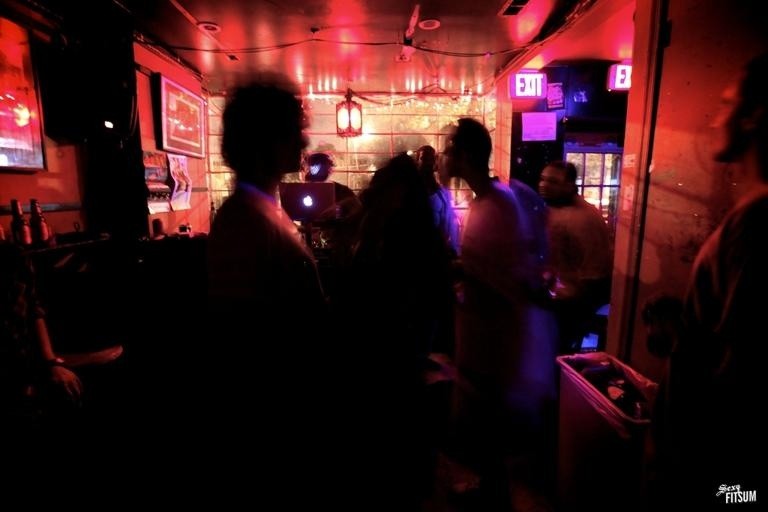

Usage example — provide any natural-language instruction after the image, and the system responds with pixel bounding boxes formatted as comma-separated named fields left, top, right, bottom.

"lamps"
left=336, top=87, right=363, bottom=137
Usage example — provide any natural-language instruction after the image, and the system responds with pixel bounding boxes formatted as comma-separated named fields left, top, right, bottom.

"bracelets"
left=50, top=357, right=70, bottom=368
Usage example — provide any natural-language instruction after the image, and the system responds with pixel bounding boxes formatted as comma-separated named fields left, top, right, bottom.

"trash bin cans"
left=555, top=352, right=660, bottom=510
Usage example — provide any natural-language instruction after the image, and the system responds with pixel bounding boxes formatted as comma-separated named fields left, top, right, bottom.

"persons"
left=641, top=51, right=766, bottom=511
left=31, top=229, right=130, bottom=407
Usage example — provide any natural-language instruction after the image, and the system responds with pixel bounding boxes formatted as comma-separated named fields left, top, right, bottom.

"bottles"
left=9, top=199, right=33, bottom=247
left=29, top=198, right=50, bottom=246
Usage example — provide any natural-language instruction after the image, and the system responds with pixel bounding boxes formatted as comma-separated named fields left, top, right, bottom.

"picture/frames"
left=0, top=15, right=47, bottom=172
left=154, top=72, right=205, bottom=159
